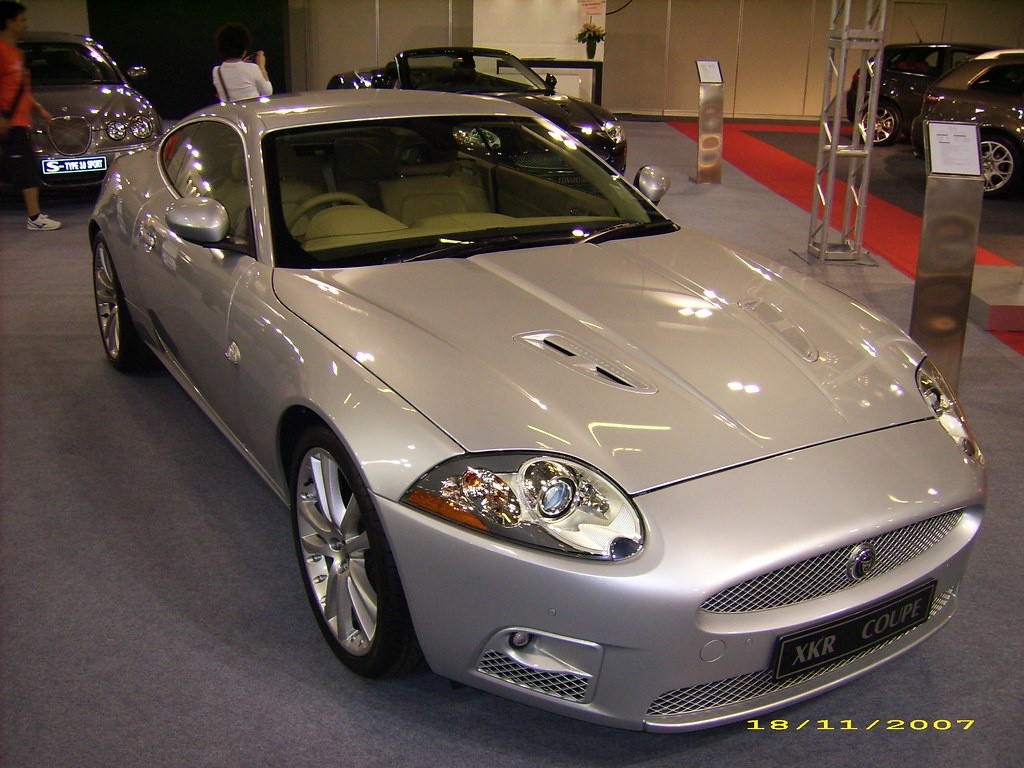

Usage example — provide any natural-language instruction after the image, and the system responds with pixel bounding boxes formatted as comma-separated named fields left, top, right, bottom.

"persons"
left=0, top=0, right=62, bottom=231
left=211, top=23, right=273, bottom=103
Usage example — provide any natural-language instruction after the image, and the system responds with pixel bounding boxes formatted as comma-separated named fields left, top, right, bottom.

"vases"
left=587, top=40, right=596, bottom=59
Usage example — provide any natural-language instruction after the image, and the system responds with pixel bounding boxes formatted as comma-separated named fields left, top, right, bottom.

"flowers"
left=574, top=23, right=606, bottom=42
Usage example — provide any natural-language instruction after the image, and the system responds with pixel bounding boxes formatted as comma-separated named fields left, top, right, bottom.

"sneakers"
left=27, top=213, right=61, bottom=230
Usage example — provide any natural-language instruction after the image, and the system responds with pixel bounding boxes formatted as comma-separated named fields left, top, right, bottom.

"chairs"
left=383, top=61, right=484, bottom=92
left=206, top=139, right=491, bottom=244
left=896, top=60, right=934, bottom=75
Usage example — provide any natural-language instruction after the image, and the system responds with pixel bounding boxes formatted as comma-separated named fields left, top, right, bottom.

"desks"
left=496, top=58, right=603, bottom=104
